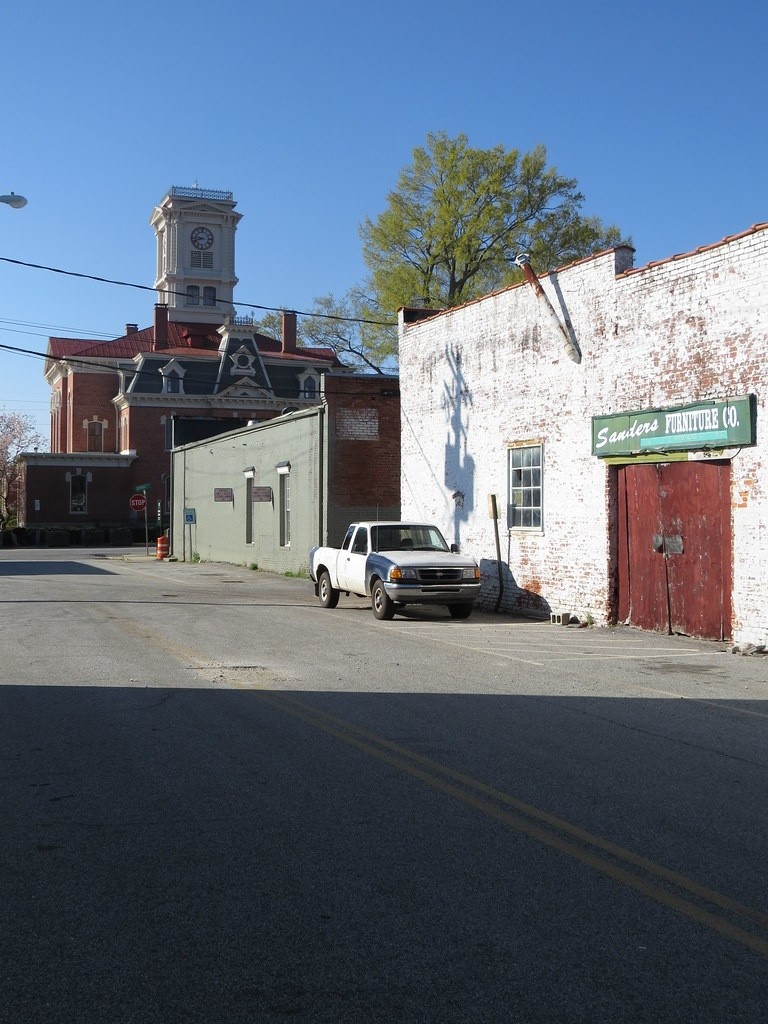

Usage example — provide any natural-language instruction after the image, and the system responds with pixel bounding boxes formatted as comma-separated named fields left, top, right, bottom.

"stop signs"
left=130, top=494, right=147, bottom=511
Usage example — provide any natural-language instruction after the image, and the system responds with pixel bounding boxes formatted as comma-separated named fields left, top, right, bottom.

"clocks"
left=190, top=226, right=214, bottom=250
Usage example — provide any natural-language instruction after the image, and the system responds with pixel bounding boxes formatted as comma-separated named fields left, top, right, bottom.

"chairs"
left=400, top=538, right=423, bottom=547
left=362, top=535, right=387, bottom=548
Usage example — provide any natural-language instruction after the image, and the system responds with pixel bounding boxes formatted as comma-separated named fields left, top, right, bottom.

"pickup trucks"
left=308, top=520, right=484, bottom=621
